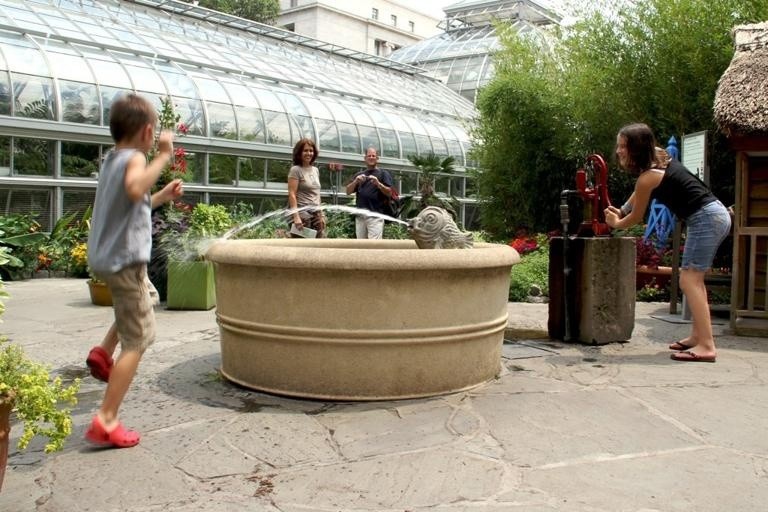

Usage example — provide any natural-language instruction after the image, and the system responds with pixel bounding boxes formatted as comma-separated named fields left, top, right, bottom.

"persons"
left=346, top=147, right=393, bottom=240
left=80, top=93, right=184, bottom=448
left=604, top=122, right=731, bottom=362
left=285, top=137, right=326, bottom=239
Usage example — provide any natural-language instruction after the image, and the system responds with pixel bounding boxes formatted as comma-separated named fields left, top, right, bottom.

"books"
left=288, top=222, right=318, bottom=239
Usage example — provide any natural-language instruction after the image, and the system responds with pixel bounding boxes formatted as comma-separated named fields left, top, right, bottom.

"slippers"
left=669, top=342, right=690, bottom=350
left=671, top=351, right=715, bottom=362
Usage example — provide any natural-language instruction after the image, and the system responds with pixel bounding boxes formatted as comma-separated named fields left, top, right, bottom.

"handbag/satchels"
left=382, top=186, right=400, bottom=224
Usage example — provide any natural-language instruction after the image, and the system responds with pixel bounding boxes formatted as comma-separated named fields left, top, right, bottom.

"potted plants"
left=168, top=201, right=234, bottom=310
left=86, top=265, right=115, bottom=307
left=0, top=341, right=80, bottom=494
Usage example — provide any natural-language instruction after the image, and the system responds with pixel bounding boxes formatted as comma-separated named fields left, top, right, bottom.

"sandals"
left=86, top=347, right=114, bottom=382
left=85, top=415, right=140, bottom=447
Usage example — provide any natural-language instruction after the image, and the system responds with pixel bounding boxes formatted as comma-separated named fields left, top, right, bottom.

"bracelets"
left=621, top=202, right=632, bottom=214
